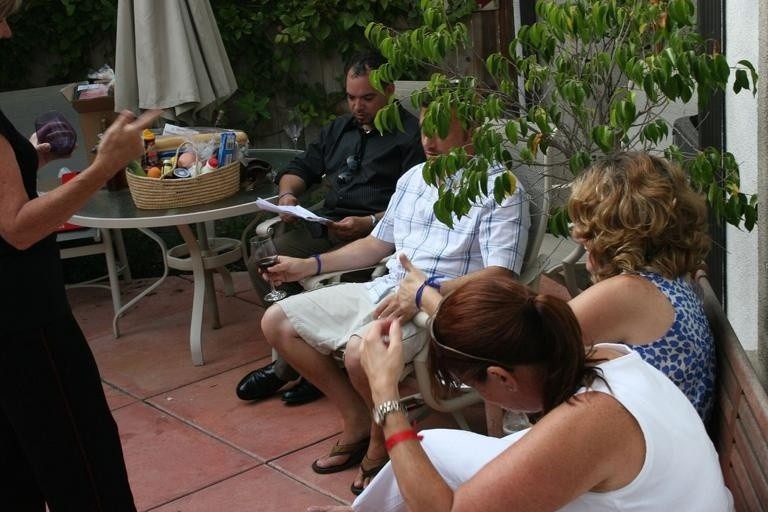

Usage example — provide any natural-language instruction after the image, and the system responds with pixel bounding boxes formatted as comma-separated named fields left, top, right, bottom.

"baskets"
left=126, top=162, right=240, bottom=211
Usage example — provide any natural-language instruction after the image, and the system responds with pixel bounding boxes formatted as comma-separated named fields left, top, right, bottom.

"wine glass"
left=36, top=109, right=82, bottom=176
left=247, top=233, right=286, bottom=302
left=281, top=105, right=304, bottom=156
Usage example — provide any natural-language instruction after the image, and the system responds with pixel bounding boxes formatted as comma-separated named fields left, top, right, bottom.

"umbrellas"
left=112, top=0, right=241, bottom=328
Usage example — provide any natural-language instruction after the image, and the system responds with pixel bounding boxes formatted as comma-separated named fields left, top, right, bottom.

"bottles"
left=201, top=158, right=218, bottom=176
left=161, top=159, right=174, bottom=178
left=501, top=407, right=528, bottom=435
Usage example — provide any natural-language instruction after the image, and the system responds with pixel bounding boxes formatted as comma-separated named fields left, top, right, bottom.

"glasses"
left=427, top=291, right=513, bottom=372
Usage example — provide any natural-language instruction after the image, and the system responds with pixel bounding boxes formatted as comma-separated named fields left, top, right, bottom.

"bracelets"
left=370, top=214, right=377, bottom=231
left=415, top=279, right=439, bottom=309
left=278, top=192, right=294, bottom=201
left=312, top=253, right=323, bottom=276
left=385, top=430, right=418, bottom=455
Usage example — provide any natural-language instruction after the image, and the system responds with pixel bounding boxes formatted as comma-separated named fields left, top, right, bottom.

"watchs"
left=371, top=400, right=408, bottom=424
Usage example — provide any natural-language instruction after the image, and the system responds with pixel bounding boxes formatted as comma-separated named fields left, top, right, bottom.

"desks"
left=36, top=148, right=324, bottom=367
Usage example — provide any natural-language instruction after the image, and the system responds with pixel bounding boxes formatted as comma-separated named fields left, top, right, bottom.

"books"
left=254, top=197, right=334, bottom=227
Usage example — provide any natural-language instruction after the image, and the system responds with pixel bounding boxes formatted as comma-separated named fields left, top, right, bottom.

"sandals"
left=312, top=438, right=368, bottom=472
left=351, top=453, right=388, bottom=493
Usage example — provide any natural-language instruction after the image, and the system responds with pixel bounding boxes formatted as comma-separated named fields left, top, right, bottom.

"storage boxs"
left=59, top=77, right=119, bottom=189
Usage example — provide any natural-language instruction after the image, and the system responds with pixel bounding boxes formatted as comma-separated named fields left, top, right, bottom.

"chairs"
left=0, top=80, right=132, bottom=318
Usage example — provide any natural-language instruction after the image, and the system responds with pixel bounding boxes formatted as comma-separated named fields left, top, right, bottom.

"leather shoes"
left=277, top=371, right=325, bottom=404
left=237, top=360, right=288, bottom=401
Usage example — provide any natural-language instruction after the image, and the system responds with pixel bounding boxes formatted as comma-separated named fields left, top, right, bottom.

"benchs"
left=485, top=258, right=768, bottom=512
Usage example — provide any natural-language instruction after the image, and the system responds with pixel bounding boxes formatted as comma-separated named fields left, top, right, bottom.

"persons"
left=235, top=53, right=427, bottom=405
left=258, top=88, right=533, bottom=496
left=0, top=0, right=165, bottom=512
left=394, top=144, right=720, bottom=424
left=348, top=276, right=735, bottom=511
